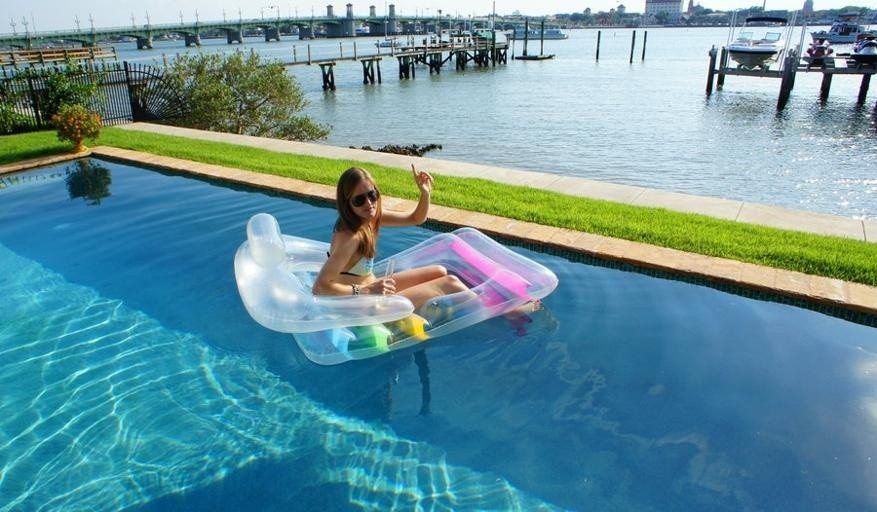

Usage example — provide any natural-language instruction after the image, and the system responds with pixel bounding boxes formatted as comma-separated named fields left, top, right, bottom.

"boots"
left=471, top=28, right=496, bottom=45
left=441, top=29, right=464, bottom=47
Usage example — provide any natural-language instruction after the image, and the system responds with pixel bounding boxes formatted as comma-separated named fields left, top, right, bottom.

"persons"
left=312, top=162, right=541, bottom=321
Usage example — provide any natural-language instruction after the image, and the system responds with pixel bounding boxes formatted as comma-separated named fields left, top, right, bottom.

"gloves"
left=809, top=21, right=876, bottom=45
left=514, top=54, right=555, bottom=61
left=849, top=33, right=876, bottom=64
left=855, top=33, right=876, bottom=43
left=373, top=41, right=402, bottom=48
left=725, top=17, right=788, bottom=72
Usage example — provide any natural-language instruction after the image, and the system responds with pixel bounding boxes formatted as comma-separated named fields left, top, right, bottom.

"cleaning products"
left=806, top=40, right=833, bottom=72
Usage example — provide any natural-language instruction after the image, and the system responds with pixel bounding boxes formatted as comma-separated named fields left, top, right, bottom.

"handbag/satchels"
left=349, top=284, right=360, bottom=298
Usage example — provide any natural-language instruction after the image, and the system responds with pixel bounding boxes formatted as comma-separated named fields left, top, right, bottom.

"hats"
left=40, top=42, right=55, bottom=50
left=449, top=32, right=472, bottom=49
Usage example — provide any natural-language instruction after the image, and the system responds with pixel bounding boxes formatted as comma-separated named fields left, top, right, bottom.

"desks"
left=436, top=10, right=441, bottom=49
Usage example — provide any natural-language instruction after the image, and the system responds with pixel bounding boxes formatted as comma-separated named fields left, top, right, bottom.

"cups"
left=352, top=191, right=379, bottom=207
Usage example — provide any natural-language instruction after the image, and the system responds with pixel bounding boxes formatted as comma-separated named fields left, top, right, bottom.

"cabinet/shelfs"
left=376, top=258, right=396, bottom=312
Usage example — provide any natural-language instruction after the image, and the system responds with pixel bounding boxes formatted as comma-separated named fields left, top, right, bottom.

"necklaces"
left=487, top=0, right=508, bottom=49
